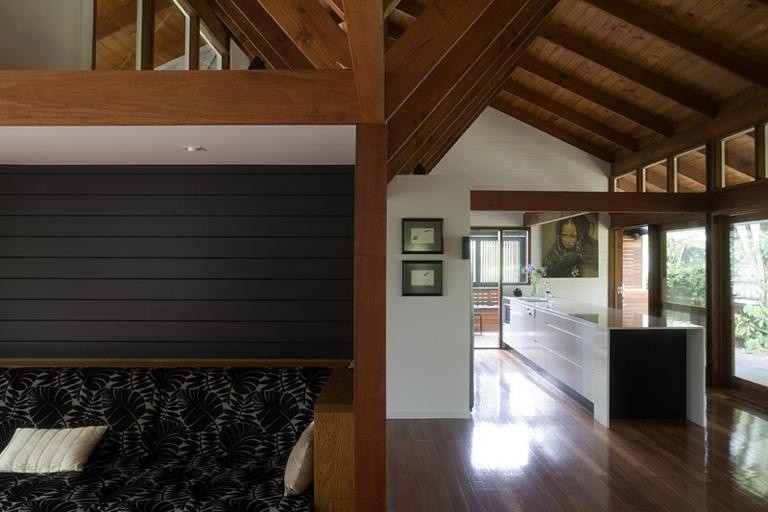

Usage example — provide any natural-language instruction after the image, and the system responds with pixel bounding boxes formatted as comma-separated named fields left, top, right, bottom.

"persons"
left=544, top=216, right=598, bottom=277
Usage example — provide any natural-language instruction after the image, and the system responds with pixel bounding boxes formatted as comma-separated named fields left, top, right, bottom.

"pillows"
left=283, top=420, right=314, bottom=497
left=0, top=424, right=109, bottom=476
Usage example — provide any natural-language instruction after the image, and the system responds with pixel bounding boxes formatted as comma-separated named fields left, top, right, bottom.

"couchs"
left=0, top=356, right=356, bottom=511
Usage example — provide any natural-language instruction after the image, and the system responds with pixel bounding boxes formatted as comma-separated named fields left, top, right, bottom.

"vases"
left=529, top=274, right=539, bottom=296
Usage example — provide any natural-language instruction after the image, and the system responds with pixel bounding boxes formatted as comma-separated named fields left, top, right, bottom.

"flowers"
left=524, top=264, right=547, bottom=296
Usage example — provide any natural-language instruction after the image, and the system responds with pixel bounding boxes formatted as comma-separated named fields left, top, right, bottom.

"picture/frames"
left=402, top=217, right=444, bottom=255
left=401, top=260, right=443, bottom=297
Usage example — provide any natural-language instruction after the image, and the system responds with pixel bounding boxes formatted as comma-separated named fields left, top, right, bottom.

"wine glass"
left=543, top=282, right=552, bottom=308
